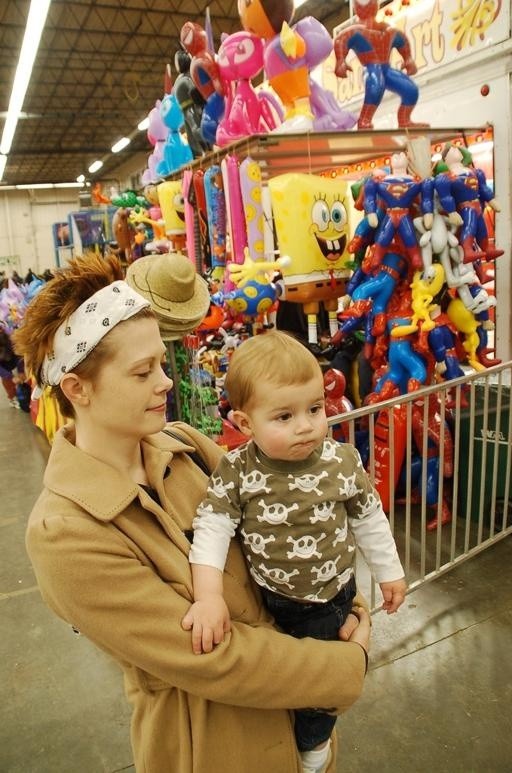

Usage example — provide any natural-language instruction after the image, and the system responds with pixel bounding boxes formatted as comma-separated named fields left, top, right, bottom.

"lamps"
left=77, top=119, right=150, bottom=183
left=0, top=0, right=51, bottom=183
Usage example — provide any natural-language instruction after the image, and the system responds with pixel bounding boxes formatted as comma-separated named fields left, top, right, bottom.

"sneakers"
left=301, top=748, right=333, bottom=772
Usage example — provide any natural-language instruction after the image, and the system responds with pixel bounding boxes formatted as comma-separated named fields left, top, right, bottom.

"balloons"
left=87, top=0, right=506, bottom=532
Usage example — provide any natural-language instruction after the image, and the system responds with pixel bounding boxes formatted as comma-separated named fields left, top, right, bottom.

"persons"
left=179, top=330, right=409, bottom=773
left=238, top=0, right=314, bottom=133
left=8, top=240, right=372, bottom=773
left=322, top=146, right=506, bottom=532
left=335, top=0, right=430, bottom=130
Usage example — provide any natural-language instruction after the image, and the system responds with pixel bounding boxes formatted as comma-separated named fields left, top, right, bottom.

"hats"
left=125, top=253, right=211, bottom=343
